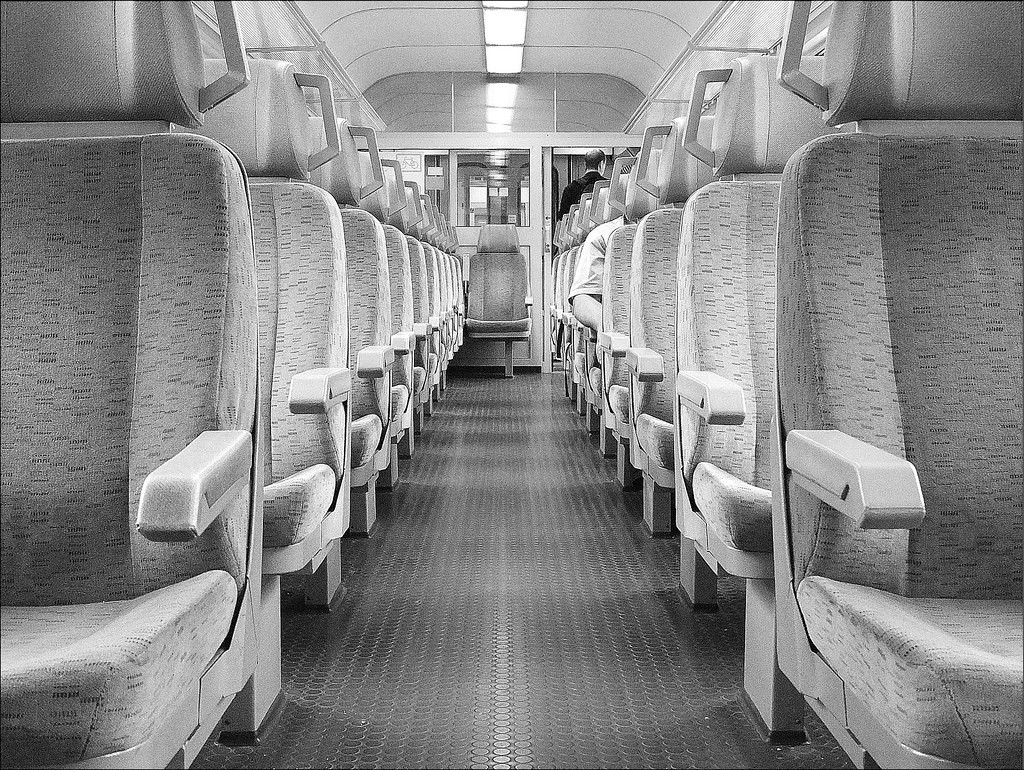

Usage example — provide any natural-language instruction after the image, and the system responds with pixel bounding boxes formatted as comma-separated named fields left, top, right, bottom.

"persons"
left=568, top=213, right=636, bottom=334
left=559, top=149, right=611, bottom=220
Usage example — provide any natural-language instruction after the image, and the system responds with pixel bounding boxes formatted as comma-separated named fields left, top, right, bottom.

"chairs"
left=0, top=0, right=1024, bottom=770
